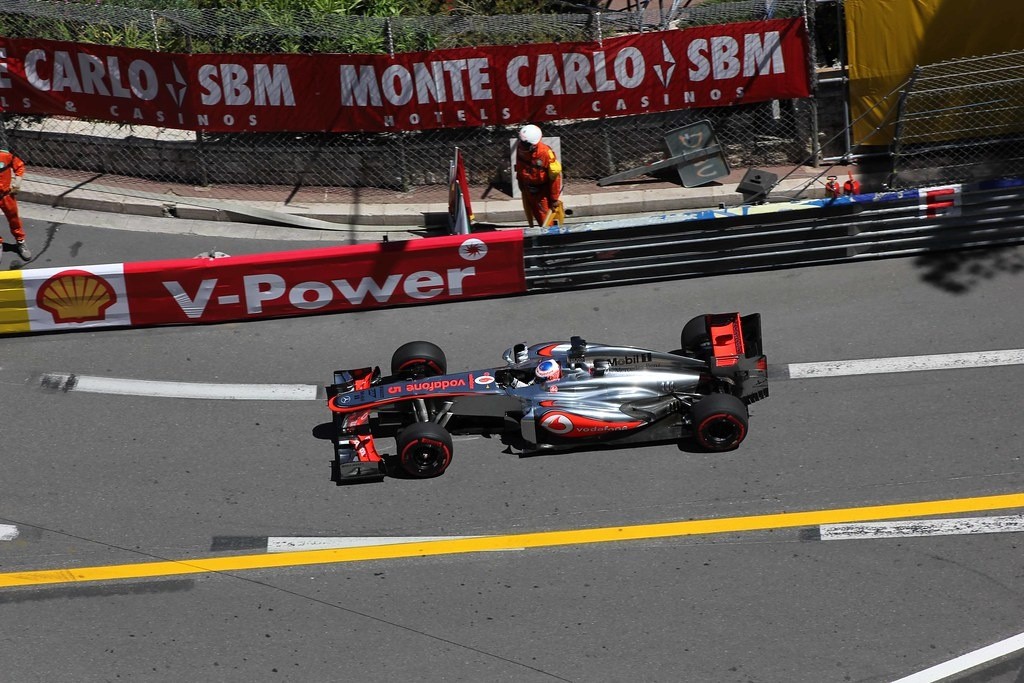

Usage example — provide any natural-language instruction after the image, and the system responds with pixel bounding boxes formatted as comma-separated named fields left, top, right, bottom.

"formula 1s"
left=320, top=309, right=773, bottom=486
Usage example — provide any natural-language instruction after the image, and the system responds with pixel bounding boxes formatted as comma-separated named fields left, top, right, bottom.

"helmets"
left=519, top=124, right=542, bottom=145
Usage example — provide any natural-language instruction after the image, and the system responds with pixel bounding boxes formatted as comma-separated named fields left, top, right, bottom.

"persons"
left=516, top=125, right=561, bottom=226
left=529, top=359, right=560, bottom=385
left=0, top=149, right=32, bottom=260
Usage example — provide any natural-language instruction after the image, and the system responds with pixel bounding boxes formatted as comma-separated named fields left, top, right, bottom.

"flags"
left=449, top=151, right=475, bottom=235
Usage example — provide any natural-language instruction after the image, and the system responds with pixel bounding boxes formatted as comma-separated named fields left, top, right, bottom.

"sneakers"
left=17, top=239, right=31, bottom=260
left=0, top=243, right=3, bottom=263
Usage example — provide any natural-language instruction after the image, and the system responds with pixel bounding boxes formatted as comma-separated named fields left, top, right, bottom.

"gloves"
left=548, top=200, right=559, bottom=213
left=10, top=176, right=21, bottom=196
